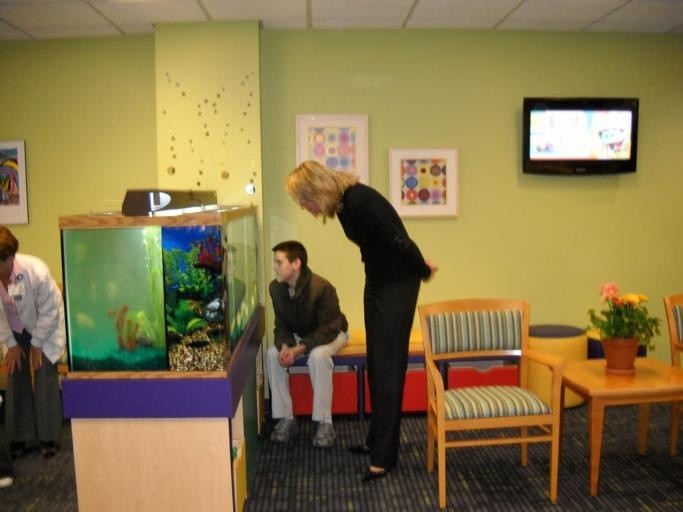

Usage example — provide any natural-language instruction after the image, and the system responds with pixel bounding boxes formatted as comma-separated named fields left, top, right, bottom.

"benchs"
left=263, top=332, right=521, bottom=417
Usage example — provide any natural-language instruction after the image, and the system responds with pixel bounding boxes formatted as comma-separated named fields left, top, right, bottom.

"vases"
left=601, top=338, right=639, bottom=376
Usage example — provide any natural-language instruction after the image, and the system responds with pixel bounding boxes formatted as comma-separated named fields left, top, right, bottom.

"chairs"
left=663, top=294, right=683, bottom=458
left=418, top=298, right=564, bottom=509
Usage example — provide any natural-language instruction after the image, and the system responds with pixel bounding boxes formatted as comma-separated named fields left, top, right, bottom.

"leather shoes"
left=348, top=443, right=371, bottom=454
left=362, top=465, right=392, bottom=482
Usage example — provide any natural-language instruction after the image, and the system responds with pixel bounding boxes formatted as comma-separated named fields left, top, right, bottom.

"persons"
left=285, top=161, right=439, bottom=484
left=0, top=224, right=25, bottom=486
left=0, top=253, right=68, bottom=458
left=263, top=240, right=350, bottom=448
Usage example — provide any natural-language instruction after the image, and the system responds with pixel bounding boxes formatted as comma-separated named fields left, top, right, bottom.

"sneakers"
left=39, top=443, right=57, bottom=456
left=313, top=424, right=338, bottom=449
left=270, top=415, right=299, bottom=442
left=9, top=444, right=27, bottom=458
left=0, top=464, right=15, bottom=489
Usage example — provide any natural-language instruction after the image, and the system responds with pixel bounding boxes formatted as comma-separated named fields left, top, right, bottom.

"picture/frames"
left=387, top=147, right=459, bottom=219
left=0, top=140, right=29, bottom=227
left=295, top=113, right=370, bottom=188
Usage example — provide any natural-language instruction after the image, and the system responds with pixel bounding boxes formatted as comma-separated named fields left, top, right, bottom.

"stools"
left=526, top=325, right=588, bottom=409
left=587, top=327, right=647, bottom=360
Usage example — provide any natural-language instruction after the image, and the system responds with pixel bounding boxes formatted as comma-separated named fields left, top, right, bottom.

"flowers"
left=585, top=281, right=660, bottom=351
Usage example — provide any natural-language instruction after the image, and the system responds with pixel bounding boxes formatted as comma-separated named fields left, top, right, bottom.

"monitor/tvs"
left=522, top=96, right=641, bottom=174
